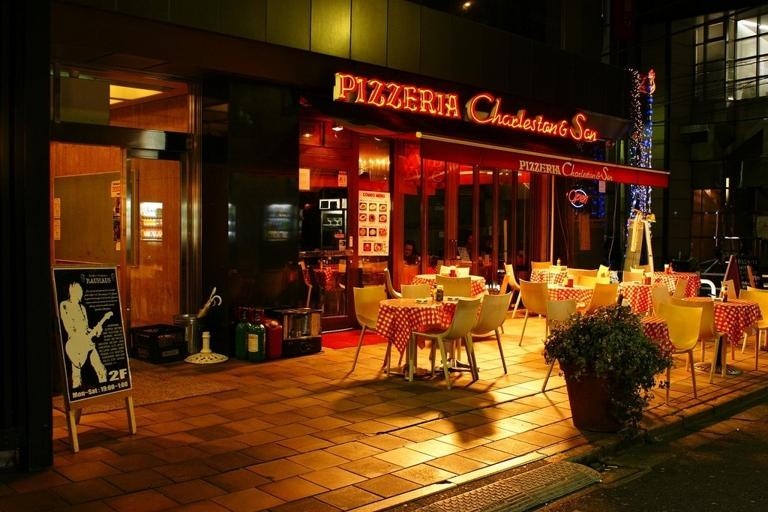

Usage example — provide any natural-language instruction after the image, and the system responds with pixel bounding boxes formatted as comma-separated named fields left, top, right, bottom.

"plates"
left=359, top=204, right=387, bottom=251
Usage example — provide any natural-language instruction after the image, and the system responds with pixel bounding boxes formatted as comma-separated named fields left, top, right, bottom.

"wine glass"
left=326, top=217, right=342, bottom=225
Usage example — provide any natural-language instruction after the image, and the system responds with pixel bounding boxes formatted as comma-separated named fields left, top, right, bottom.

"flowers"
left=544, top=305, right=675, bottom=436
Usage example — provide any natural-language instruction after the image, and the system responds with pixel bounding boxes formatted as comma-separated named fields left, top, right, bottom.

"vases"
left=559, top=352, right=618, bottom=430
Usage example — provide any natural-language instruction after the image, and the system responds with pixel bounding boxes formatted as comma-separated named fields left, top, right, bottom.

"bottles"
left=272, top=207, right=288, bottom=216
left=720, top=284, right=727, bottom=302
left=430, top=278, right=444, bottom=302
left=449, top=269, right=457, bottom=277
left=665, top=263, right=672, bottom=274
left=272, top=219, right=287, bottom=228
left=562, top=278, right=573, bottom=287
left=228, top=306, right=266, bottom=358
left=642, top=272, right=651, bottom=284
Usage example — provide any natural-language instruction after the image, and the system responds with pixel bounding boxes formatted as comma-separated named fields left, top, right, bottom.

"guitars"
left=66, top=312, right=113, bottom=368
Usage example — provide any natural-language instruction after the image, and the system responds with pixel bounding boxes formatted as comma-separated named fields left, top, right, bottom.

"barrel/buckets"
left=176, top=312, right=205, bottom=353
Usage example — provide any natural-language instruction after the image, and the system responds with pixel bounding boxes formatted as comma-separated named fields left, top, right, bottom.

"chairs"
left=299, top=257, right=346, bottom=315
left=346, top=265, right=514, bottom=391
left=504, top=261, right=768, bottom=404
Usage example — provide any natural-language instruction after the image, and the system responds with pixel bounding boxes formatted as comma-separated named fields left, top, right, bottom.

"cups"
left=271, top=232, right=288, bottom=239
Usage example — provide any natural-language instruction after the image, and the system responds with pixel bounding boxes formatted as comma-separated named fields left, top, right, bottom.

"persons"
left=404, top=239, right=421, bottom=265
left=463, top=233, right=472, bottom=259
